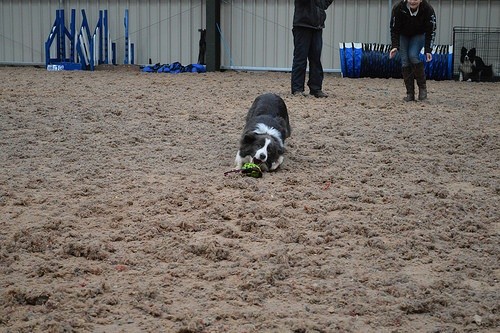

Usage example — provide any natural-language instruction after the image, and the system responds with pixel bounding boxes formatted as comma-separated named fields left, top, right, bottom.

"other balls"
left=241, top=160, right=263, bottom=180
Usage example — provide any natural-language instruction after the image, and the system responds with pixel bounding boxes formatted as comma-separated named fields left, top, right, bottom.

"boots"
left=402, top=65, right=415, bottom=101
left=413, top=61, right=429, bottom=99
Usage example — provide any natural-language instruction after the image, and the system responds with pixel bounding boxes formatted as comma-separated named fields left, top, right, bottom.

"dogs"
left=458, top=46, right=493, bottom=82
left=235, top=93, right=291, bottom=173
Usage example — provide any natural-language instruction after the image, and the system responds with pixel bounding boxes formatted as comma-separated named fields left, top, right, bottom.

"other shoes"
left=309, top=89, right=328, bottom=98
left=294, top=91, right=306, bottom=98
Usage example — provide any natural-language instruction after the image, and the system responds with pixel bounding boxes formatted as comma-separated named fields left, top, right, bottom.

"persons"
left=387, top=0, right=438, bottom=104
left=291, top=0, right=333, bottom=98
